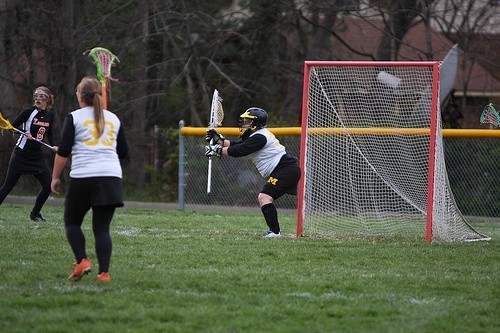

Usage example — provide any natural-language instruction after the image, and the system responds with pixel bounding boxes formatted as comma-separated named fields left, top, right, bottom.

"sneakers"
left=262, top=231, right=281, bottom=238
left=69, top=259, right=93, bottom=282
left=29, top=212, right=45, bottom=221
left=96, top=272, right=110, bottom=282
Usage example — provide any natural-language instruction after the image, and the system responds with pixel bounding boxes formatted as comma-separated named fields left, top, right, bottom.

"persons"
left=206, top=108, right=301, bottom=238
left=0, top=87, right=59, bottom=223
left=50, top=77, right=124, bottom=283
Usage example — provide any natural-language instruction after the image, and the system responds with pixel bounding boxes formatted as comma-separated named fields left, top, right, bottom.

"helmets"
left=240, top=108, right=268, bottom=130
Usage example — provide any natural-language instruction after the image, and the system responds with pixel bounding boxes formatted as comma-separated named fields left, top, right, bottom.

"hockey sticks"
left=479, top=104, right=500, bottom=129
left=88, top=47, right=115, bottom=111
left=207, top=88, right=224, bottom=193
left=0, top=113, right=55, bottom=151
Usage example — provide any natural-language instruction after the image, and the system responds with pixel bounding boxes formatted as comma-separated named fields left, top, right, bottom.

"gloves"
left=204, top=146, right=221, bottom=159
left=205, top=129, right=224, bottom=145
